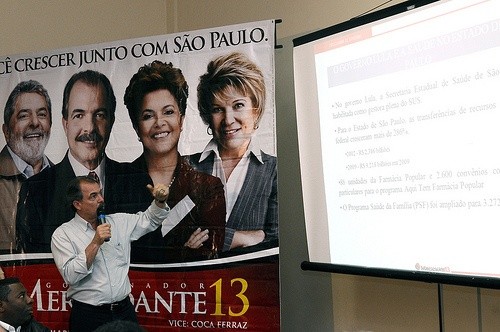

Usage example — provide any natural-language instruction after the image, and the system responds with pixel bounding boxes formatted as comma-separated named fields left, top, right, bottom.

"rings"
left=161, top=189, right=165, bottom=192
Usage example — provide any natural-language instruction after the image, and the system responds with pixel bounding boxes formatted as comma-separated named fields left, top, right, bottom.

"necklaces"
left=167, top=176, right=176, bottom=188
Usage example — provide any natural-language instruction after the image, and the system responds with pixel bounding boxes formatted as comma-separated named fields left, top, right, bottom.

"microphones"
left=97, top=207, right=110, bottom=243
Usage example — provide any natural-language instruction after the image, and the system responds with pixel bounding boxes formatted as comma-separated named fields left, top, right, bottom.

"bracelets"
left=155, top=198, right=167, bottom=204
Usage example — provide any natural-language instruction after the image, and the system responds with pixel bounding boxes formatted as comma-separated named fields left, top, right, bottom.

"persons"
left=15, top=70, right=121, bottom=253
left=108, top=60, right=227, bottom=264
left=0, top=278, right=51, bottom=332
left=51, top=176, right=170, bottom=332
left=181, top=52, right=279, bottom=258
left=0, top=80, right=55, bottom=255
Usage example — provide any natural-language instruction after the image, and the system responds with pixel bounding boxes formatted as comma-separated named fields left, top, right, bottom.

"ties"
left=89, top=172, right=99, bottom=182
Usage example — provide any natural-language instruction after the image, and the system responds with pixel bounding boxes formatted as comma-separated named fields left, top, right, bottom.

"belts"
left=73, top=297, right=128, bottom=311
